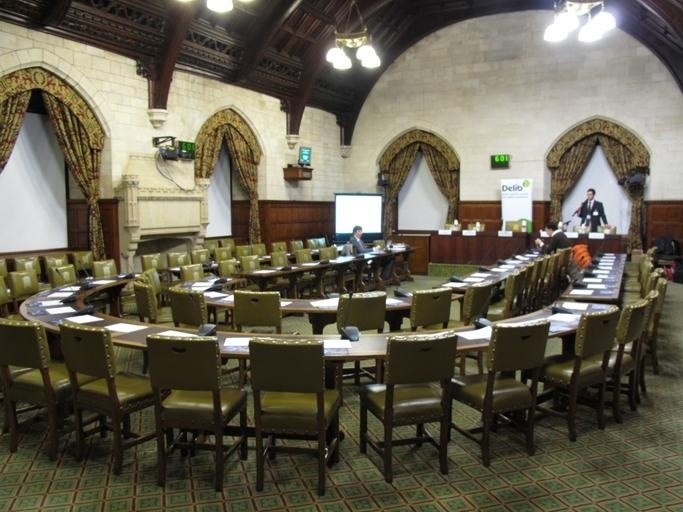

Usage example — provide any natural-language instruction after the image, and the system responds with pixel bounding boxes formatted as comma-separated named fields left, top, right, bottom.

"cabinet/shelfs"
left=283, top=167, right=313, bottom=180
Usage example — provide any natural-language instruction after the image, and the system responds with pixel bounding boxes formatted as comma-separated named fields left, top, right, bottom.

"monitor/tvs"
left=298, top=146, right=311, bottom=168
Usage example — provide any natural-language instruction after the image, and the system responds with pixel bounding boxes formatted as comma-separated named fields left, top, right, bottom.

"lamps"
left=325, top=1, right=382, bottom=71
left=176, top=0, right=258, bottom=14
left=543, top=0, right=619, bottom=47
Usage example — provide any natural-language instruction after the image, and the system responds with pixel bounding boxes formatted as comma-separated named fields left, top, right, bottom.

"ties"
left=588, top=202, right=591, bottom=211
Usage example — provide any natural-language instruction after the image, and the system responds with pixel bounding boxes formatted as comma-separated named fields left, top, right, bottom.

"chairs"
left=249, top=336, right=342, bottom=495
left=145, top=334, right=248, bottom=491
left=0, top=320, right=107, bottom=463
left=522, top=307, right=619, bottom=444
left=551, top=298, right=648, bottom=424
left=358, top=329, right=462, bottom=483
left=453, top=317, right=552, bottom=470
left=56, top=322, right=176, bottom=474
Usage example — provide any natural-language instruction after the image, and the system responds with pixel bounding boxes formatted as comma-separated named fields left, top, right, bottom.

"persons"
left=533, top=222, right=570, bottom=255
left=347, top=225, right=394, bottom=281
left=576, top=188, right=608, bottom=234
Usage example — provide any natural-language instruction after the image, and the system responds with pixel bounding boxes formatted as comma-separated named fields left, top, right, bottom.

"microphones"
left=472, top=287, right=498, bottom=328
left=78, top=260, right=94, bottom=290
left=395, top=244, right=606, bottom=298
left=55, top=267, right=77, bottom=304
left=188, top=293, right=217, bottom=336
left=341, top=291, right=360, bottom=341
left=552, top=278, right=579, bottom=315
left=121, top=253, right=135, bottom=279
left=176, top=233, right=416, bottom=290
left=572, top=211, right=578, bottom=216
left=73, top=267, right=94, bottom=315
left=563, top=220, right=571, bottom=225
